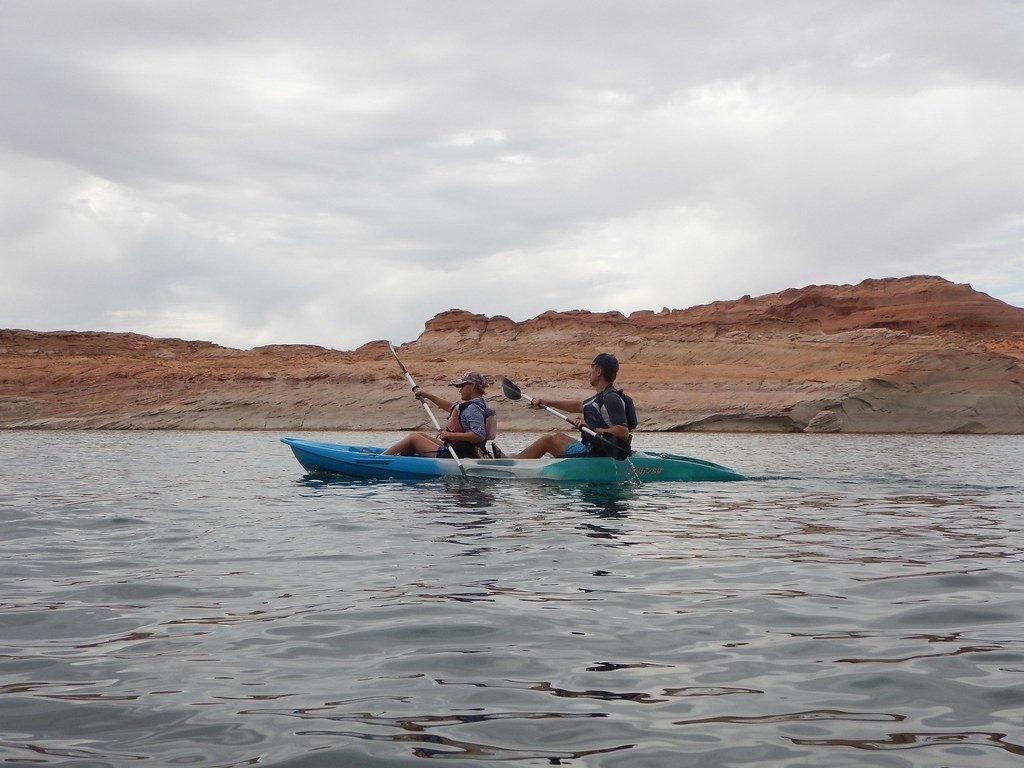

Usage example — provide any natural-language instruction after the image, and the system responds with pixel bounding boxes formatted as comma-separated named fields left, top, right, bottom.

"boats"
left=280, top=435, right=750, bottom=483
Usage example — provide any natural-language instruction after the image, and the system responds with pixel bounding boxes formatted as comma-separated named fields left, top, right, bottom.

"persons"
left=475, top=353, right=629, bottom=462
left=361, top=371, right=488, bottom=459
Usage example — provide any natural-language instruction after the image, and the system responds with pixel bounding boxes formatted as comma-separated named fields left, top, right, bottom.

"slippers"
left=475, top=447, right=486, bottom=459
left=492, top=442, right=501, bottom=459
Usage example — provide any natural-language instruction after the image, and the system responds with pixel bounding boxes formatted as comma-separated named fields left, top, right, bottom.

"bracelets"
left=589, top=426, right=596, bottom=431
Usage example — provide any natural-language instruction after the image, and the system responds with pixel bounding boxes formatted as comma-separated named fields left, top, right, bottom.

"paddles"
left=387, top=340, right=470, bottom=485
left=500, top=376, right=632, bottom=462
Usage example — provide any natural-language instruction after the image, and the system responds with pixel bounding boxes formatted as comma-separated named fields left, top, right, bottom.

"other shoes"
left=362, top=450, right=370, bottom=454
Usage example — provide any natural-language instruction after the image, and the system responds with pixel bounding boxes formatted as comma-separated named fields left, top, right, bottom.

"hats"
left=451, top=371, right=487, bottom=390
left=590, top=353, right=618, bottom=369
